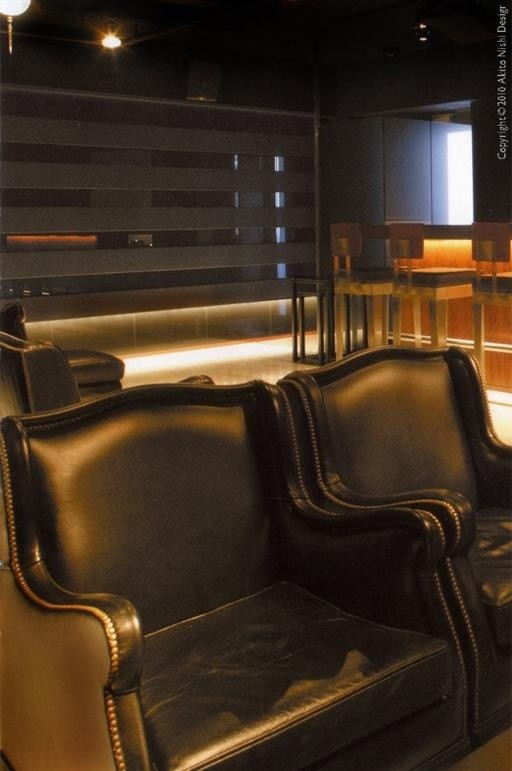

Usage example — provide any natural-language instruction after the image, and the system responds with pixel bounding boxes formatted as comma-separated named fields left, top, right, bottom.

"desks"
left=288, top=272, right=370, bottom=366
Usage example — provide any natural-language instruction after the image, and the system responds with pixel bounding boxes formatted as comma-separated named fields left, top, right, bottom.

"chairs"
left=329, top=223, right=510, bottom=405
left=0, top=298, right=509, bottom=770
